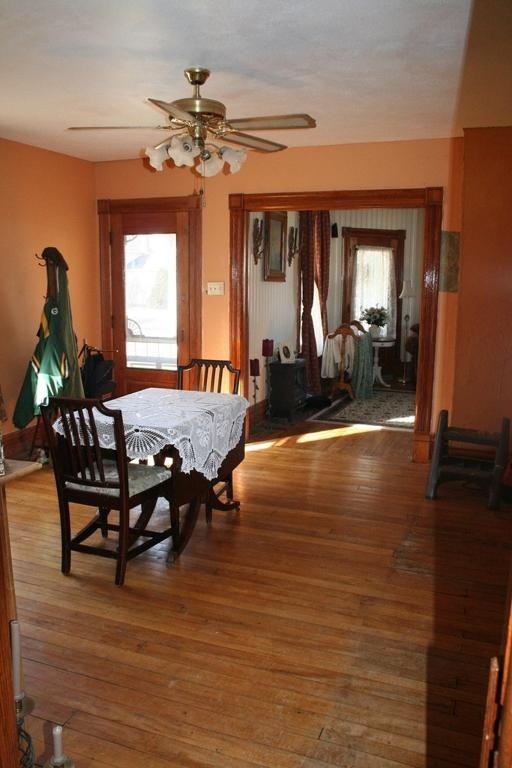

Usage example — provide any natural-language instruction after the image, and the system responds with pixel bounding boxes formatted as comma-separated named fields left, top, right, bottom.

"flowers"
left=361, top=304, right=389, bottom=326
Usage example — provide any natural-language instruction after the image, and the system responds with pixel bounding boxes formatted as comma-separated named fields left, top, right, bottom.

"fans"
left=66, top=68, right=317, bottom=154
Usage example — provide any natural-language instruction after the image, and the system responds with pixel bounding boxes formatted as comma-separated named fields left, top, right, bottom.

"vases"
left=369, top=325, right=380, bottom=337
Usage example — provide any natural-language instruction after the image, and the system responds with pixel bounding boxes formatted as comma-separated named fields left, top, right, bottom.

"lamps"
left=146, top=133, right=245, bottom=178
left=398, top=280, right=416, bottom=383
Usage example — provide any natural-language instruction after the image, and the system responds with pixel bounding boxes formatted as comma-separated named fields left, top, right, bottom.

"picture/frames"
left=263, top=211, right=288, bottom=283
left=277, top=341, right=295, bottom=363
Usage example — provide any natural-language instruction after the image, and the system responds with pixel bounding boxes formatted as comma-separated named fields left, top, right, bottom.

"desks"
left=53, top=387, right=250, bottom=559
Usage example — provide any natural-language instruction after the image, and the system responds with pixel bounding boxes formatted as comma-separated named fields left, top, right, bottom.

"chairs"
left=41, top=396, right=180, bottom=587
left=177, top=359, right=240, bottom=526
left=426, top=410, right=510, bottom=509
